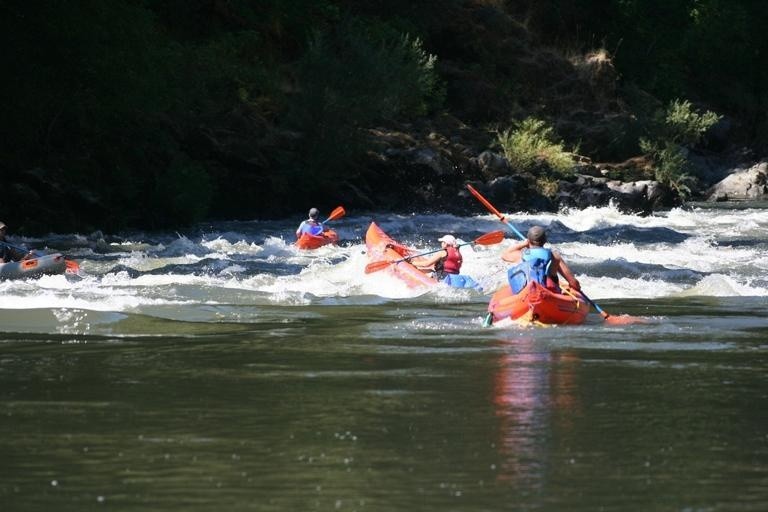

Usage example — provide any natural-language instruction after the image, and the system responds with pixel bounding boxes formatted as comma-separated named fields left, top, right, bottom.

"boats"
left=292, top=226, right=338, bottom=250
left=481, top=280, right=590, bottom=328
left=0, top=249, right=78, bottom=280
left=365, top=221, right=483, bottom=298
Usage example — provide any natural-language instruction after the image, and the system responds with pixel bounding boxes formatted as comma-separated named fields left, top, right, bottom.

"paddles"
left=364, top=229, right=503, bottom=273
left=465, top=183, right=645, bottom=325
left=322, top=207, right=346, bottom=224
left=0, top=239, right=78, bottom=270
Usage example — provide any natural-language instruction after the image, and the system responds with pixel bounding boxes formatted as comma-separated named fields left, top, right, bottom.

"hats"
left=0, top=221, right=8, bottom=230
left=527, top=226, right=546, bottom=244
left=438, top=235, right=457, bottom=245
left=309, top=207, right=319, bottom=217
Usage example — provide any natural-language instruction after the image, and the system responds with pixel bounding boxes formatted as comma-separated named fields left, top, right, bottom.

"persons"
left=295, top=206, right=329, bottom=239
left=0, top=221, right=34, bottom=263
left=499, top=224, right=582, bottom=295
left=409, top=234, right=463, bottom=281
